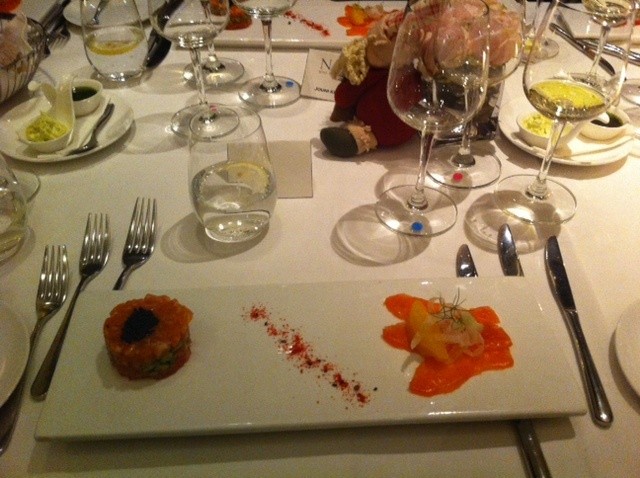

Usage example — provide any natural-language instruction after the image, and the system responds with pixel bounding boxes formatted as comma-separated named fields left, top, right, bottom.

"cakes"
left=104, top=294, right=193, bottom=383
left=200, top=0, right=253, bottom=31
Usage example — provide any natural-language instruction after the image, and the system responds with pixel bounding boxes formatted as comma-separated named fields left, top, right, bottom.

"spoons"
left=26, top=78, right=103, bottom=117
left=515, top=110, right=610, bottom=149
left=66, top=103, right=114, bottom=156
left=16, top=71, right=78, bottom=151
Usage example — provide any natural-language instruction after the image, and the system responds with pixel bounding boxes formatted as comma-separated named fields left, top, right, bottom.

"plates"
left=33, top=276, right=588, bottom=441
left=62, top=0, right=167, bottom=29
left=0, top=90, right=134, bottom=162
left=614, top=296, right=640, bottom=398
left=557, top=3, right=632, bottom=40
left=163, top=0, right=409, bottom=50
left=0, top=299, right=31, bottom=409
left=497, top=95, right=634, bottom=167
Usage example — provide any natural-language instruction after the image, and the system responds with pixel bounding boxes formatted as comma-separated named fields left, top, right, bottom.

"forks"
left=112, top=197, right=158, bottom=290
left=0, top=243, right=69, bottom=455
left=51, top=20, right=70, bottom=51
left=30, top=212, right=111, bottom=401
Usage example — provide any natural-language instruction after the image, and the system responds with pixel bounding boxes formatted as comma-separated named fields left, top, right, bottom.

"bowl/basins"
left=580, top=106, right=630, bottom=140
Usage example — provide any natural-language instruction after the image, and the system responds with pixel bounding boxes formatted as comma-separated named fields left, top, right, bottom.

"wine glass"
left=519, top=1, right=560, bottom=65
left=375, top=0, right=491, bottom=236
left=231, top=0, right=301, bottom=108
left=147, top=0, right=240, bottom=140
left=182, top=38, right=245, bottom=85
left=567, top=0, right=640, bottom=107
left=494, top=0, right=638, bottom=224
left=426, top=0, right=525, bottom=189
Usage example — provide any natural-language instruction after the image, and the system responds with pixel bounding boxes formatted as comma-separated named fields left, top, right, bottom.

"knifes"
left=456, top=244, right=552, bottom=478
left=545, top=235, right=614, bottom=427
left=497, top=223, right=524, bottom=276
left=549, top=23, right=615, bottom=75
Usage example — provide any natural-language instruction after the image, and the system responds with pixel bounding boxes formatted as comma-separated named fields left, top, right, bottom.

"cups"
left=188, top=103, right=278, bottom=243
left=0, top=153, right=25, bottom=251
left=80, top=0, right=146, bottom=84
